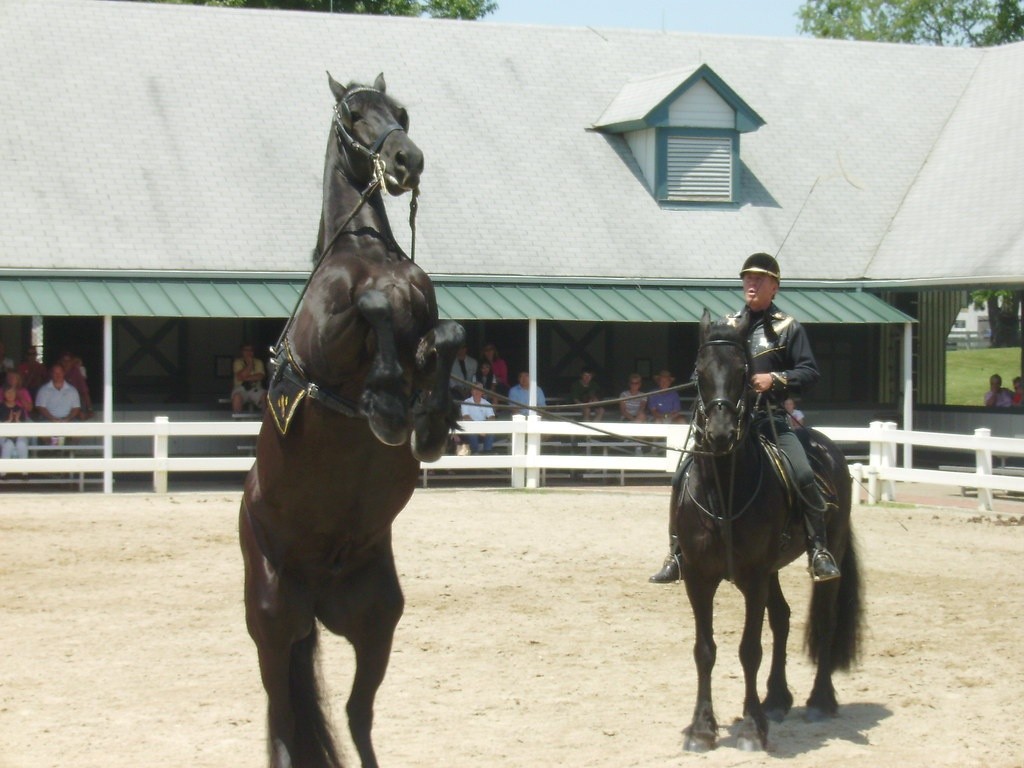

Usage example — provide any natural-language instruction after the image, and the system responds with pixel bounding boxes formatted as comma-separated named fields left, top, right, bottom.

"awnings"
left=0, top=274, right=919, bottom=494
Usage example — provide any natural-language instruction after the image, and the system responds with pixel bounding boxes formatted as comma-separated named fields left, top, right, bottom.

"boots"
left=648, top=514, right=684, bottom=583
left=799, top=483, right=840, bottom=581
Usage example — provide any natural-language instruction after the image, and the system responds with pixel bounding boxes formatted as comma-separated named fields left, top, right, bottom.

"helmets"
left=740, top=253, right=780, bottom=285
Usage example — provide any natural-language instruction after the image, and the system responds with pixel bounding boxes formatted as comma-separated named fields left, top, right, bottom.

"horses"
left=237, top=69, right=469, bottom=768
left=669, top=306, right=876, bottom=753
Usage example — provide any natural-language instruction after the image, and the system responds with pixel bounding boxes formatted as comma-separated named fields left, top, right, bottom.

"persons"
left=460, top=383, right=496, bottom=454
left=648, top=252, right=840, bottom=584
left=618, top=373, right=647, bottom=456
left=647, top=369, right=688, bottom=454
left=447, top=343, right=508, bottom=411
left=983, top=373, right=1015, bottom=407
left=1012, top=376, right=1022, bottom=404
left=506, top=370, right=546, bottom=417
left=0, top=342, right=92, bottom=459
left=569, top=368, right=605, bottom=423
left=784, top=397, right=804, bottom=426
left=230, top=344, right=267, bottom=416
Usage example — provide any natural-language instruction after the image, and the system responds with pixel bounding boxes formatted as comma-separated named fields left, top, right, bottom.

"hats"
left=654, top=370, right=675, bottom=383
